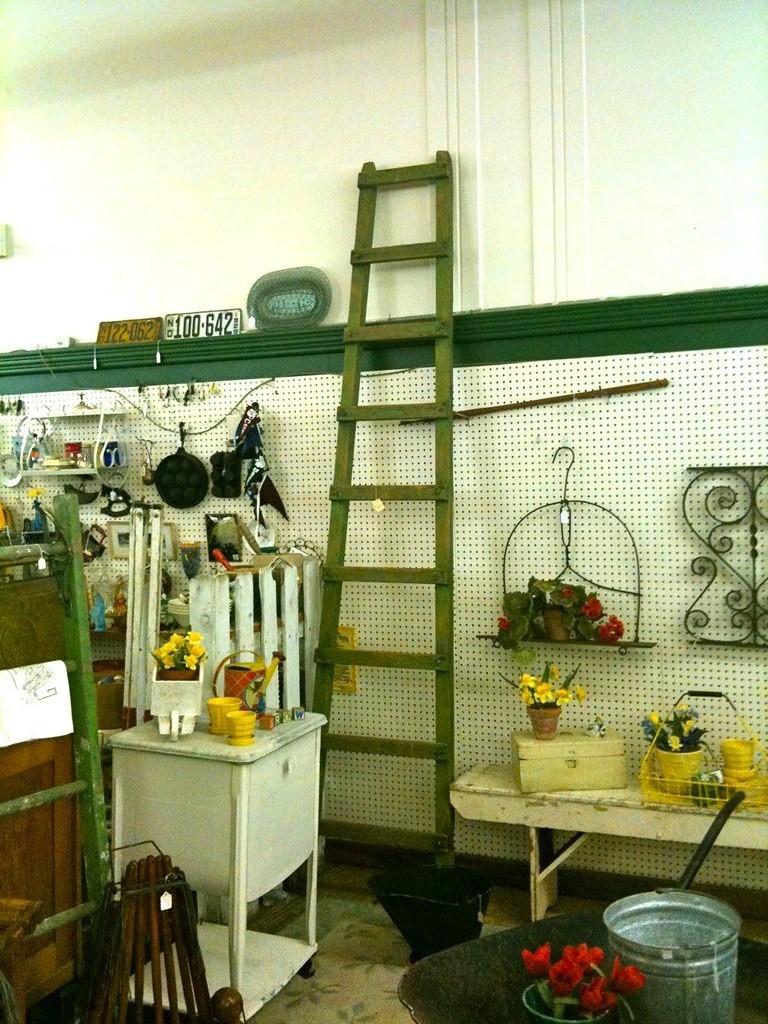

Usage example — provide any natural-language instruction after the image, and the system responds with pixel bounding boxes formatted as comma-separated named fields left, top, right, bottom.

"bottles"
left=64, top=439, right=121, bottom=467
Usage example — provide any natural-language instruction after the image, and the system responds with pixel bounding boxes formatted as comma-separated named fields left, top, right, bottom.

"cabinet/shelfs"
left=109, top=712, right=326, bottom=1023
left=638, top=692, right=767, bottom=817
left=472, top=446, right=654, bottom=654
left=89, top=613, right=307, bottom=753
left=18, top=405, right=124, bottom=477
left=0, top=736, right=87, bottom=1022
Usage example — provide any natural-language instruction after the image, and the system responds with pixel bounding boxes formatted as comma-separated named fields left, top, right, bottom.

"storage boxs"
left=513, top=730, right=625, bottom=793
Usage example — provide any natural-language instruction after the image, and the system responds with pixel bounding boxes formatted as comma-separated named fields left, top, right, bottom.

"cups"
left=720, top=739, right=753, bottom=771
left=225, top=711, right=257, bottom=739
left=207, top=696, right=242, bottom=727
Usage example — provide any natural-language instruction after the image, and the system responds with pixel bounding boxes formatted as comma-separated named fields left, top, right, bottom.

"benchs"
left=451, top=759, right=767, bottom=923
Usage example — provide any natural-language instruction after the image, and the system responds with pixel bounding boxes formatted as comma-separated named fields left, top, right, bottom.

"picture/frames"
left=205, top=514, right=241, bottom=562
left=332, top=626, right=357, bottom=696
left=107, top=520, right=177, bottom=561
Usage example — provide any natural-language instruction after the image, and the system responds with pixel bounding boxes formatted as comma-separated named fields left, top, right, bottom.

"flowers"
left=496, top=577, right=622, bottom=652
left=524, top=944, right=643, bottom=1017
left=500, top=664, right=586, bottom=709
left=642, top=701, right=705, bottom=752
left=151, top=630, right=208, bottom=672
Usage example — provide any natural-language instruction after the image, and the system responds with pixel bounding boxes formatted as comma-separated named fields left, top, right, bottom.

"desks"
left=398, top=903, right=767, bottom=1024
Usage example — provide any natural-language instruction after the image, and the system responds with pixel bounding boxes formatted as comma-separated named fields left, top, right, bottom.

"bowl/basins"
left=167, top=597, right=233, bottom=629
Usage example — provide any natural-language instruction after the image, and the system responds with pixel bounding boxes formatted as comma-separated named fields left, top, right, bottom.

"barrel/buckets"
left=602, top=886, right=742, bottom=1024
left=369, top=865, right=493, bottom=964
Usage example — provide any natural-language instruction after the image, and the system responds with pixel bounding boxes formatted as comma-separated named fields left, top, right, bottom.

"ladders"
left=284, top=151, right=454, bottom=884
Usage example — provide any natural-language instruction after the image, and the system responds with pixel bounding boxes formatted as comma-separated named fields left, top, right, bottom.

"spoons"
left=141, top=440, right=155, bottom=486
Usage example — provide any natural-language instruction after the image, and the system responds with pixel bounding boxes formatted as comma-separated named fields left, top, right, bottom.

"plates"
left=243, top=517, right=276, bottom=556
left=0, top=453, right=24, bottom=488
left=722, top=764, right=757, bottom=781
left=208, top=723, right=229, bottom=734
left=228, top=734, right=255, bottom=746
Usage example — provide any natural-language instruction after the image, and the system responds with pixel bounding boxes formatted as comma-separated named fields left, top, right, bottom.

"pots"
left=155, top=421, right=209, bottom=509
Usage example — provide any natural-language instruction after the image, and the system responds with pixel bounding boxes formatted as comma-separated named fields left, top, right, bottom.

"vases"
left=150, top=665, right=203, bottom=735
left=652, top=746, right=701, bottom=794
left=539, top=607, right=576, bottom=643
left=524, top=984, right=618, bottom=1023
left=721, top=741, right=756, bottom=779
left=527, top=703, right=560, bottom=740
